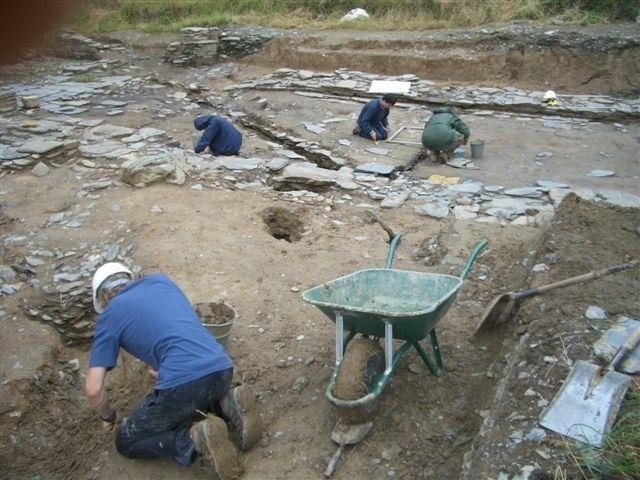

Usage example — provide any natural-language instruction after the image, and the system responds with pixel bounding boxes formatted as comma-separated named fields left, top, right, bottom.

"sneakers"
left=195, top=416, right=242, bottom=480
left=352, top=127, right=361, bottom=135
left=227, top=383, right=262, bottom=452
left=439, top=152, right=448, bottom=164
left=429, top=151, right=438, bottom=162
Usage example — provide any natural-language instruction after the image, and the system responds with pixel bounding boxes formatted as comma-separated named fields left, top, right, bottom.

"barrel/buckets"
left=192, top=131, right=203, bottom=147
left=192, top=301, right=239, bottom=350
left=471, top=139, right=484, bottom=158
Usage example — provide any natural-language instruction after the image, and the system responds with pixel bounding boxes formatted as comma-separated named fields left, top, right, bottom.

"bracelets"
left=100, top=409, right=117, bottom=422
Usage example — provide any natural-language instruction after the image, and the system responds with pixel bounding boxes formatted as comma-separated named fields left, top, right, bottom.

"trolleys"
left=300, top=232, right=489, bottom=420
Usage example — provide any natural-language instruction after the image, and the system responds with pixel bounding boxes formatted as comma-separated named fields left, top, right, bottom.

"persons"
left=85, top=261, right=263, bottom=479
left=421, top=107, right=470, bottom=162
left=193, top=115, right=243, bottom=157
left=352, top=93, right=398, bottom=140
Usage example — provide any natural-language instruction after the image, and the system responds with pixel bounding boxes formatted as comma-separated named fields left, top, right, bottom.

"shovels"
left=537, top=325, right=640, bottom=450
left=474, top=260, right=639, bottom=336
left=324, top=415, right=374, bottom=478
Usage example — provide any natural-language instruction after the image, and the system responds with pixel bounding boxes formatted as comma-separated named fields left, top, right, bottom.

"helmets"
left=92, top=262, right=135, bottom=314
